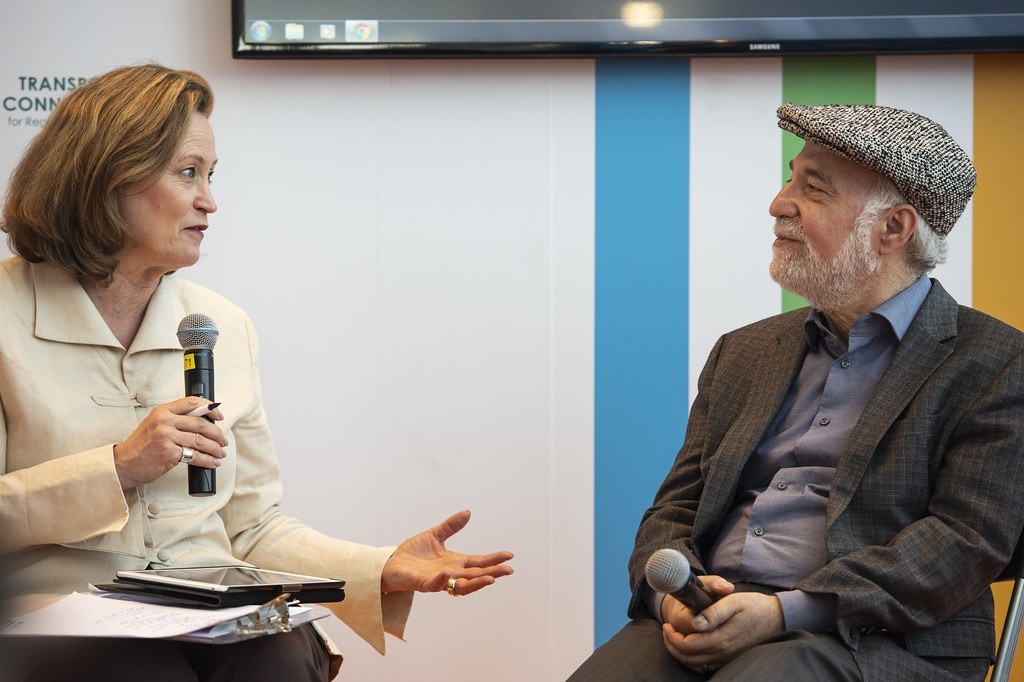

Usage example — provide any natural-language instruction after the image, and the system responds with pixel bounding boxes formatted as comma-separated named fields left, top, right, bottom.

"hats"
left=779, top=101, right=975, bottom=237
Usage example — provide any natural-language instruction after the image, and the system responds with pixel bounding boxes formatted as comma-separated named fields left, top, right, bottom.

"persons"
left=0, top=63, right=515, bottom=682
left=564, top=102, right=1024, bottom=682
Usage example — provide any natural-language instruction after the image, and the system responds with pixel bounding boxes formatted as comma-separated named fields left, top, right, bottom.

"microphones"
left=176, top=313, right=219, bottom=497
left=645, top=549, right=729, bottom=626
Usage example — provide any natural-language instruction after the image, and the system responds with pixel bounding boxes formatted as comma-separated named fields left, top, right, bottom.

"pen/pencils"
left=185, top=402, right=221, bottom=417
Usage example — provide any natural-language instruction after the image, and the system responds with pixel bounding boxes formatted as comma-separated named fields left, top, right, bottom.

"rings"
left=178, top=447, right=193, bottom=463
left=445, top=576, right=462, bottom=597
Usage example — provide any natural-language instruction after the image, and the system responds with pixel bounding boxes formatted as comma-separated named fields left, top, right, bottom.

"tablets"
left=116, top=565, right=346, bottom=596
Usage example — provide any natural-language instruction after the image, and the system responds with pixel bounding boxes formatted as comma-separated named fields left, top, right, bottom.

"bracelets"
left=382, top=592, right=388, bottom=594
